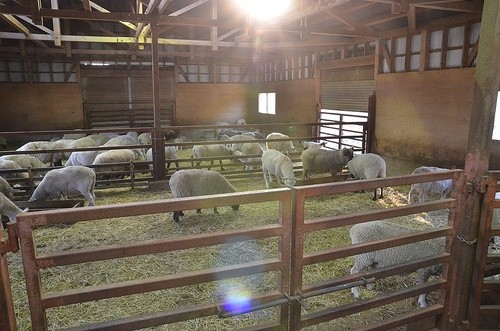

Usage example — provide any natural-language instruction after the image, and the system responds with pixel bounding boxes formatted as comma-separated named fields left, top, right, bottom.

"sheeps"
left=0, top=190, right=29, bottom=223
left=64, top=149, right=103, bottom=165
left=260, top=148, right=301, bottom=188
left=0, top=154, right=49, bottom=177
left=169, top=169, right=240, bottom=223
left=145, top=146, right=180, bottom=176
left=424, top=192, right=500, bottom=229
left=138, top=133, right=166, bottom=157
left=348, top=220, right=445, bottom=309
left=138, top=133, right=152, bottom=144
left=219, top=129, right=243, bottom=137
left=63, top=132, right=120, bottom=157
left=0, top=175, right=14, bottom=201
left=300, top=144, right=354, bottom=180
left=300, top=141, right=328, bottom=150
left=405, top=165, right=453, bottom=204
left=28, top=165, right=96, bottom=206
left=265, top=132, right=297, bottom=153
left=172, top=135, right=186, bottom=151
left=0, top=158, right=36, bottom=191
left=192, top=117, right=265, bottom=169
left=49, top=139, right=76, bottom=166
left=16, top=141, right=53, bottom=163
left=345, top=153, right=386, bottom=202
left=126, top=131, right=138, bottom=139
left=91, top=149, right=136, bottom=186
left=97, top=135, right=144, bottom=159
